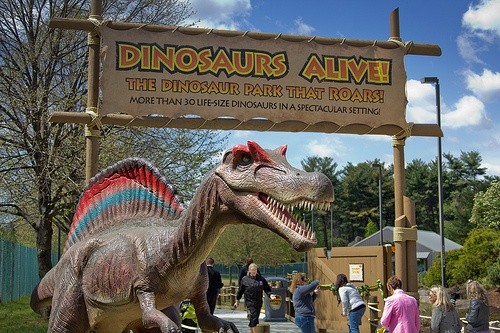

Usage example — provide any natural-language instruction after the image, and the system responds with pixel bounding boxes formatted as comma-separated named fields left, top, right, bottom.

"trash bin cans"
left=262, top=277, right=289, bottom=322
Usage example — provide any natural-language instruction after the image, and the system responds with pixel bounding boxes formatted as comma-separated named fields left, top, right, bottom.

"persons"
left=234, top=258, right=276, bottom=327
left=380, top=275, right=421, bottom=333
left=336, top=274, right=366, bottom=333
left=429, top=285, right=462, bottom=333
left=289, top=274, right=320, bottom=333
left=465, top=280, right=489, bottom=333
left=206, top=258, right=224, bottom=315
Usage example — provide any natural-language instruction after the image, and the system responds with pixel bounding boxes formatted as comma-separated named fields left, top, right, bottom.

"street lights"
left=421, top=76, right=446, bottom=287
left=372, top=164, right=383, bottom=245
